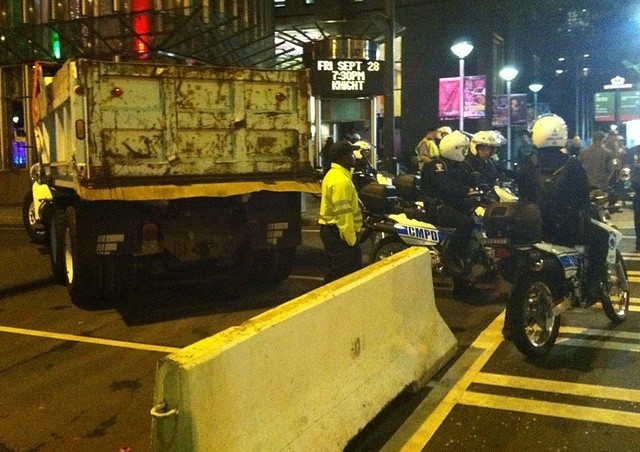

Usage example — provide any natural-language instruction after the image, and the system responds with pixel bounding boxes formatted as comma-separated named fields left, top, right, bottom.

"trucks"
left=23, top=58, right=320, bottom=301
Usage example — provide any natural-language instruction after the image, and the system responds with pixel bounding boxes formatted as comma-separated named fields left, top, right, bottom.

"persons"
left=607, top=129, right=622, bottom=184
left=350, top=134, right=362, bottom=145
left=515, top=112, right=610, bottom=293
left=317, top=138, right=364, bottom=274
left=470, top=129, right=498, bottom=177
left=436, top=126, right=453, bottom=139
left=353, top=139, right=380, bottom=184
left=322, top=135, right=331, bottom=163
left=578, top=129, right=611, bottom=189
left=493, top=127, right=507, bottom=155
left=517, top=130, right=533, bottom=155
left=624, top=144, right=639, bottom=250
left=416, top=128, right=440, bottom=168
left=571, top=134, right=583, bottom=152
left=418, top=130, right=483, bottom=276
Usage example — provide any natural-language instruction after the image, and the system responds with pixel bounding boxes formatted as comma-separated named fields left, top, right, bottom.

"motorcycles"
left=485, top=201, right=629, bottom=358
left=371, top=187, right=518, bottom=286
left=593, top=192, right=610, bottom=223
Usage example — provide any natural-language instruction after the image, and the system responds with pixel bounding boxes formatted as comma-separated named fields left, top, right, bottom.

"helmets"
left=438, top=126, right=451, bottom=140
left=439, top=130, right=469, bottom=162
left=526, top=111, right=569, bottom=149
left=487, top=131, right=506, bottom=148
left=469, top=129, right=497, bottom=157
left=353, top=141, right=374, bottom=160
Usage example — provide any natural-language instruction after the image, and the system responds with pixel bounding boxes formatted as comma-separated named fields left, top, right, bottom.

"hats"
left=328, top=141, right=361, bottom=157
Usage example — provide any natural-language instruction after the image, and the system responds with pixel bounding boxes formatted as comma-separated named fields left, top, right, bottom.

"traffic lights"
left=49, top=31, right=71, bottom=62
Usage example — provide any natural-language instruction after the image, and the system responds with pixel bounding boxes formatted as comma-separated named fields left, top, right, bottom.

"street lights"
left=452, top=40, right=476, bottom=130
left=529, top=82, right=544, bottom=120
left=500, top=65, right=518, bottom=172
